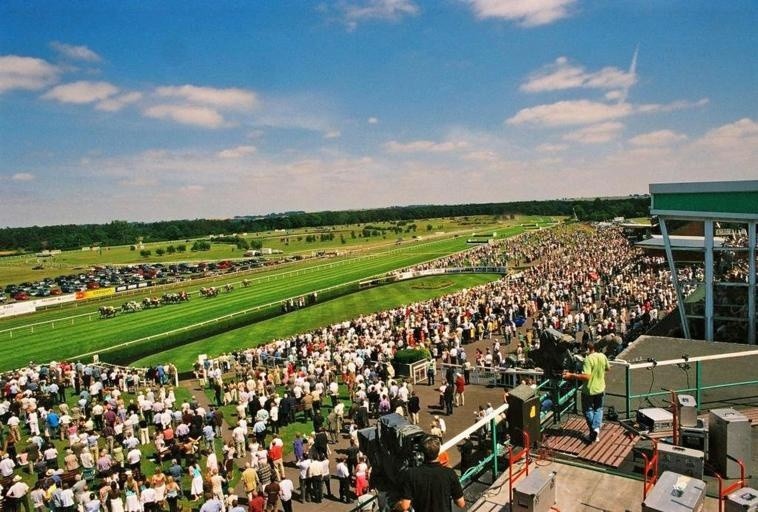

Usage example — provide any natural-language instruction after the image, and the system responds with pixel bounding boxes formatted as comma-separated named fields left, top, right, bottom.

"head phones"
left=416, top=436, right=440, bottom=463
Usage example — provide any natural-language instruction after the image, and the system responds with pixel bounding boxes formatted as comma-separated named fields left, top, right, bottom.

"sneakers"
left=590, top=429, right=599, bottom=440
left=585, top=435, right=599, bottom=442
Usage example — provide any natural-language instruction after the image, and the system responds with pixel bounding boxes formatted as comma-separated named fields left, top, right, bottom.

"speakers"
left=508, top=396, right=541, bottom=446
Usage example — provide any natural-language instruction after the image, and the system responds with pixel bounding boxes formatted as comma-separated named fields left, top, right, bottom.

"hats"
left=46, top=469, right=55, bottom=476
left=12, top=475, right=23, bottom=482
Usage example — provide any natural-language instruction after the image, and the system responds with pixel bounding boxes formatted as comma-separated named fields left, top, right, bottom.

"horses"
left=98, top=290, right=190, bottom=319
left=223, top=284, right=233, bottom=293
left=200, top=285, right=221, bottom=298
left=242, top=278, right=252, bottom=288
